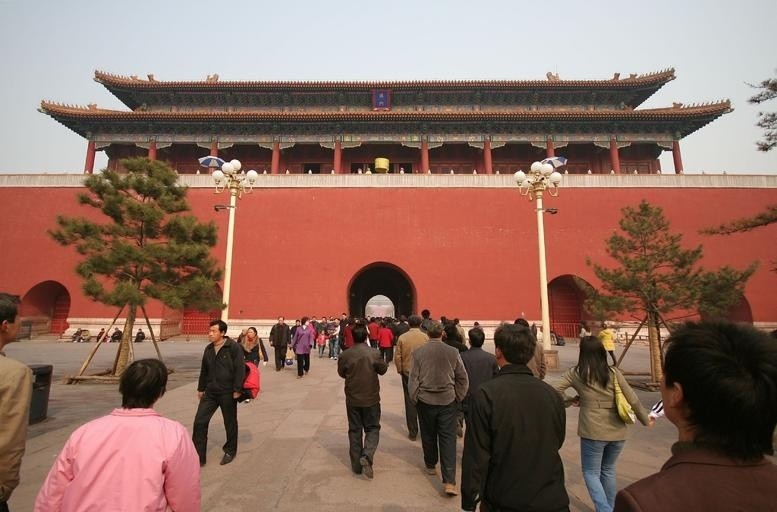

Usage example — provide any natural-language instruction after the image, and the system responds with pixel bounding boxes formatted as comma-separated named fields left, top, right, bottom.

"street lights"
left=514, top=162, right=562, bottom=372
left=212, top=159, right=258, bottom=325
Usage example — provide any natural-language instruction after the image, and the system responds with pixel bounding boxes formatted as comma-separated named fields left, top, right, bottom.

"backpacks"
left=232, top=338, right=260, bottom=403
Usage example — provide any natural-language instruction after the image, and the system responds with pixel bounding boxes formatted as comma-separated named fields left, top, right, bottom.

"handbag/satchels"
left=284, top=346, right=296, bottom=360
left=258, top=338, right=264, bottom=361
left=609, top=368, right=637, bottom=425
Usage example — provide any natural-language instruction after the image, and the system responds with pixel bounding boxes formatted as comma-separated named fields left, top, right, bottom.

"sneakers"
left=334, top=356, right=337, bottom=360
left=329, top=357, right=333, bottom=359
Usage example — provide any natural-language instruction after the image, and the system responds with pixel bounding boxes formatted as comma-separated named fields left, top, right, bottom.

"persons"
left=291, top=316, right=313, bottom=379
left=337, top=326, right=388, bottom=479
left=554, top=335, right=654, bottom=511
left=344, top=315, right=410, bottom=361
left=408, top=320, right=469, bottom=497
left=193, top=321, right=245, bottom=465
left=440, top=316, right=447, bottom=326
left=72, top=328, right=82, bottom=343
left=269, top=317, right=291, bottom=372
left=317, top=330, right=330, bottom=358
left=237, top=327, right=268, bottom=369
left=514, top=318, right=545, bottom=380
left=330, top=317, right=340, bottom=359
left=599, top=322, right=618, bottom=368
left=474, top=321, right=479, bottom=330
left=454, top=318, right=466, bottom=344
left=135, top=329, right=145, bottom=342
left=291, top=319, right=300, bottom=337
left=309, top=316, right=319, bottom=349
left=327, top=319, right=336, bottom=341
left=0, top=294, right=31, bottom=511
left=579, top=320, right=592, bottom=336
left=319, top=317, right=328, bottom=333
left=459, top=328, right=499, bottom=424
left=111, top=327, right=122, bottom=342
left=340, top=312, right=346, bottom=351
left=421, top=310, right=431, bottom=320
left=615, top=321, right=774, bottom=511
left=395, top=315, right=431, bottom=441
left=97, top=328, right=108, bottom=342
left=460, top=324, right=570, bottom=511
left=34, top=358, right=201, bottom=511
left=442, top=325, right=469, bottom=437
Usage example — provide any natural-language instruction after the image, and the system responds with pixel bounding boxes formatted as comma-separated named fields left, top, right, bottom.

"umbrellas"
left=198, top=156, right=225, bottom=175
left=541, top=156, right=568, bottom=169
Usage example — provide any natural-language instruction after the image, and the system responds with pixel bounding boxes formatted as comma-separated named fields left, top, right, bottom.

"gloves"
left=308, top=344, right=313, bottom=349
left=263, top=362, right=268, bottom=367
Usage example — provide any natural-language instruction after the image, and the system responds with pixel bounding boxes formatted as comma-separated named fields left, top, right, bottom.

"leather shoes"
left=359, top=454, right=374, bottom=479
left=219, top=452, right=235, bottom=466
left=423, top=466, right=437, bottom=476
left=443, top=482, right=461, bottom=496
